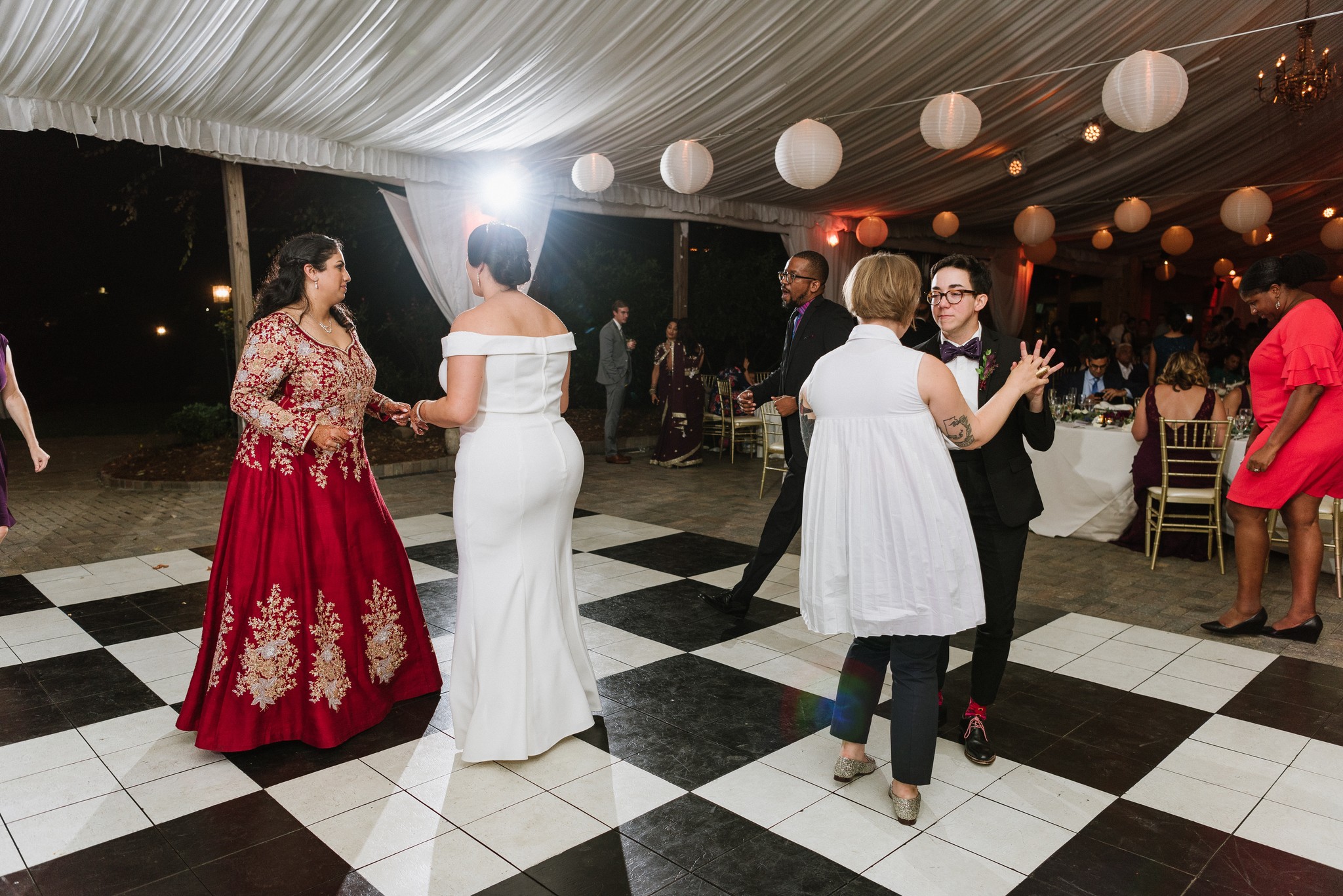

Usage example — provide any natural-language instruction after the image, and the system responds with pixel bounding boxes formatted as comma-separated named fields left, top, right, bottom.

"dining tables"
left=1020, top=404, right=1343, bottom=600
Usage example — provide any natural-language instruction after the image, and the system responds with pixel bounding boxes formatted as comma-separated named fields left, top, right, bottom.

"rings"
left=1254, top=468, right=1259, bottom=472
left=334, top=442, right=340, bottom=450
left=410, top=423, right=413, bottom=428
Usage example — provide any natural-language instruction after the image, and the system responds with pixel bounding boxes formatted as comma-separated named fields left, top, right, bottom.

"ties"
left=1092, top=379, right=1099, bottom=394
left=792, top=313, right=803, bottom=342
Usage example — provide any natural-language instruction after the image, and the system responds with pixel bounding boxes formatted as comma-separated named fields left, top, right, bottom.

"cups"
left=1134, top=397, right=1142, bottom=409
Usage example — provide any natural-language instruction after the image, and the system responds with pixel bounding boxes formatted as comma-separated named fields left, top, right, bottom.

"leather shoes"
left=960, top=709, right=996, bottom=763
left=606, top=455, right=632, bottom=462
left=610, top=454, right=629, bottom=463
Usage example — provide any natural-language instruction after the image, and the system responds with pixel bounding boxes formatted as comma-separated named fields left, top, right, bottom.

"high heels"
left=1260, top=616, right=1323, bottom=644
left=1199, top=606, right=1267, bottom=635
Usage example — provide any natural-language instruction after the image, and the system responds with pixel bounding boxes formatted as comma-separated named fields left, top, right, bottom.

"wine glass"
left=1235, top=415, right=1250, bottom=438
left=1240, top=408, right=1254, bottom=432
left=1049, top=388, right=1095, bottom=428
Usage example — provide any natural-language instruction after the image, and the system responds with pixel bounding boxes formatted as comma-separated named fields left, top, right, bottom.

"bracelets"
left=416, top=399, right=430, bottom=423
left=382, top=399, right=393, bottom=416
left=650, top=383, right=656, bottom=396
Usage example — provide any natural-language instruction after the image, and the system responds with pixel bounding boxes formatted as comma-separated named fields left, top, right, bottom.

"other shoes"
left=887, top=781, right=922, bottom=827
left=835, top=754, right=877, bottom=781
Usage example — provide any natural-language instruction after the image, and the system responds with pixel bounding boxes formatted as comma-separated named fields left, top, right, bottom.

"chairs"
left=699, top=373, right=798, bottom=501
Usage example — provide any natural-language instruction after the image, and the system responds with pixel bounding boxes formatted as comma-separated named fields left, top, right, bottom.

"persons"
left=173, top=230, right=446, bottom=755
left=708, top=352, right=756, bottom=453
left=412, top=221, right=607, bottom=766
left=595, top=298, right=637, bottom=466
left=649, top=317, right=681, bottom=428
left=901, top=252, right=1065, bottom=768
left=1197, top=252, right=1343, bottom=647
left=1128, top=349, right=1230, bottom=561
left=696, top=248, right=859, bottom=625
left=0, top=331, right=52, bottom=551
left=1018, top=287, right=1343, bottom=426
left=797, top=249, right=1051, bottom=828
left=647, top=317, right=706, bottom=468
left=748, top=371, right=756, bottom=385
left=900, top=301, right=935, bottom=350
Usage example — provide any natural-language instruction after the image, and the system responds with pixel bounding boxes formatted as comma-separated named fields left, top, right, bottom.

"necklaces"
left=298, top=300, right=332, bottom=333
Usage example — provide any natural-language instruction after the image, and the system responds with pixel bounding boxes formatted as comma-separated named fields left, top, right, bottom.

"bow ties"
left=939, top=338, right=981, bottom=363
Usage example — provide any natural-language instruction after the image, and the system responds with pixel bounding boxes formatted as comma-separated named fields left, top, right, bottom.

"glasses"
left=1088, top=361, right=1107, bottom=369
left=926, top=289, right=983, bottom=305
left=778, top=272, right=817, bottom=284
